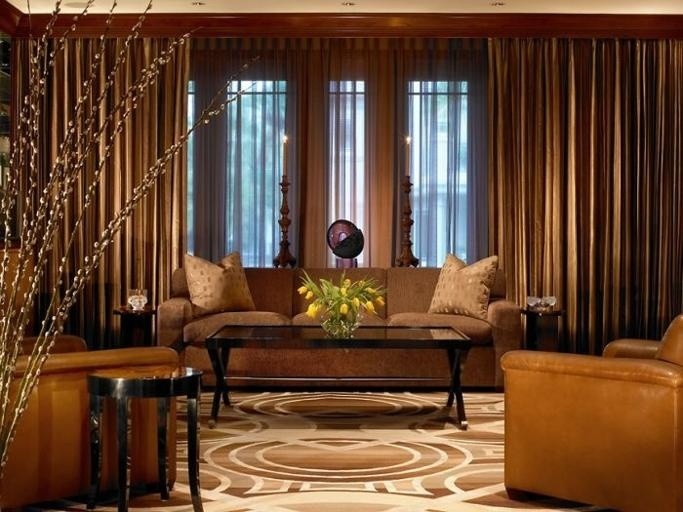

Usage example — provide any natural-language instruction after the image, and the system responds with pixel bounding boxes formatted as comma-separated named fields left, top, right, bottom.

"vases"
left=321, top=318, right=359, bottom=339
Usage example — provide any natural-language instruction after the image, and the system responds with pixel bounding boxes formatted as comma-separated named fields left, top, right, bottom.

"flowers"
left=295, top=268, right=389, bottom=323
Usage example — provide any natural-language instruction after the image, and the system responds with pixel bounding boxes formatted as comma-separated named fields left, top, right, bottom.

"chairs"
left=499, top=315, right=683, bottom=512
left=0, top=335, right=178, bottom=512
left=0, top=242, right=36, bottom=337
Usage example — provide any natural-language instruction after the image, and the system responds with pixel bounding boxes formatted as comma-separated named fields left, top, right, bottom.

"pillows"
left=184, top=253, right=256, bottom=319
left=429, top=253, right=499, bottom=322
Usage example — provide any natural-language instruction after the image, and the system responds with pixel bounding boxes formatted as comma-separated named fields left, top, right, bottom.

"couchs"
left=156, top=267, right=521, bottom=391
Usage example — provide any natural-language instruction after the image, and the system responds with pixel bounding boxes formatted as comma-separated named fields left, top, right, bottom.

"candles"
left=283, top=137, right=287, bottom=175
left=405, top=137, right=411, bottom=176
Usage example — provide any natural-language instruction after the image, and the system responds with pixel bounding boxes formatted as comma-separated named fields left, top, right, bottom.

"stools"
left=84, top=366, right=205, bottom=511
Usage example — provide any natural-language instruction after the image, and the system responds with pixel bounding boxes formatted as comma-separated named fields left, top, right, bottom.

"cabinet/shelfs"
left=0, top=0, right=33, bottom=334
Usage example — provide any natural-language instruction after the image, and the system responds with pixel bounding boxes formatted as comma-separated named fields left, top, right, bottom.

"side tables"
left=113, top=305, right=156, bottom=346
left=520, top=307, right=563, bottom=352
